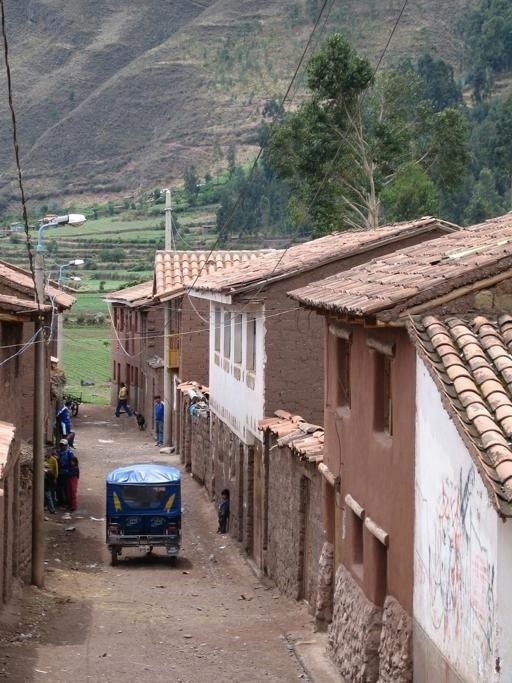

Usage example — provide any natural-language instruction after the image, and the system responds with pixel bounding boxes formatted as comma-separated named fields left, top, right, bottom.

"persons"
left=43, top=400, right=80, bottom=514
left=152, top=395, right=164, bottom=446
left=217, top=488, right=229, bottom=534
left=113, top=381, right=132, bottom=418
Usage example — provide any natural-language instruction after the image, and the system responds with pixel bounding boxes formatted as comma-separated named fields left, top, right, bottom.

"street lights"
left=156, top=186, right=175, bottom=452
left=56, top=255, right=85, bottom=374
left=32, top=212, right=87, bottom=584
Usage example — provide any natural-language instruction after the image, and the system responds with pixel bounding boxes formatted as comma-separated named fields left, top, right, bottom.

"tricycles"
left=103, top=465, right=185, bottom=567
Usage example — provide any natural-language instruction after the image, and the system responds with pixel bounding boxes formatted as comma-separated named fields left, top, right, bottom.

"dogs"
left=132, top=410, right=146, bottom=430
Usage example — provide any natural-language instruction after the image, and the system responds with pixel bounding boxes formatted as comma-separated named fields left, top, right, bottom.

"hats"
left=59, top=438, right=68, bottom=446
